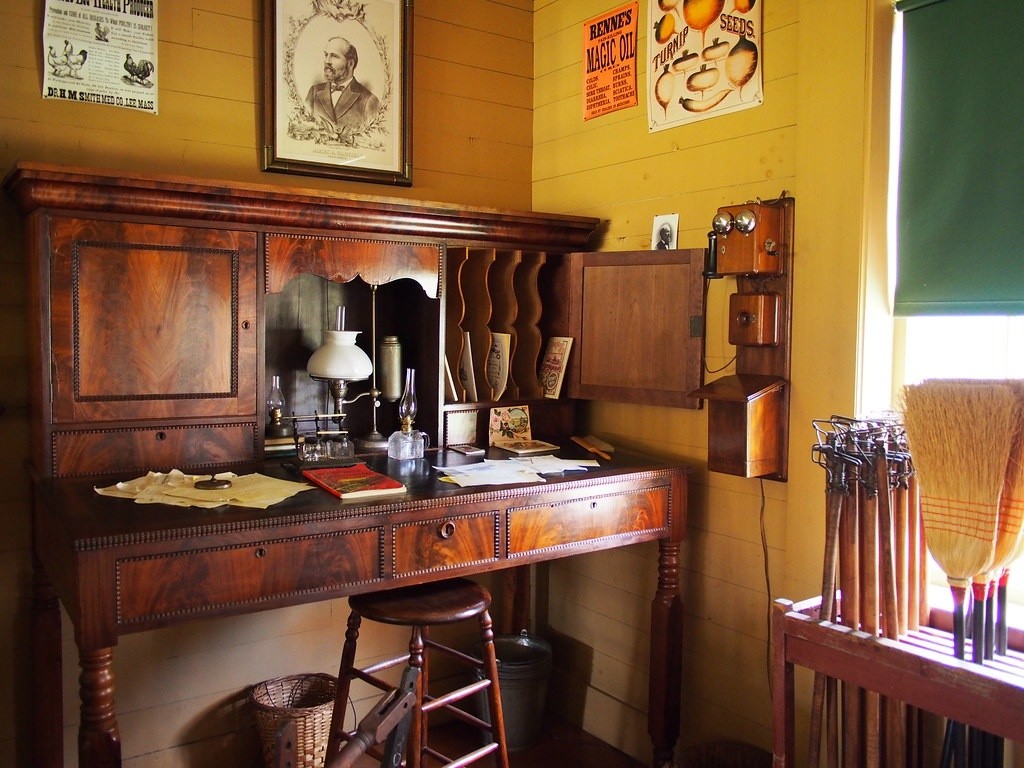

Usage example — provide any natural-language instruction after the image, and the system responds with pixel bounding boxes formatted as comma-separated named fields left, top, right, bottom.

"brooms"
left=900, top=378, right=1023, bottom=767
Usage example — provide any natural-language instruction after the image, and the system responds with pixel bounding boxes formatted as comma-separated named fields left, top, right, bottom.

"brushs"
left=569, top=433, right=615, bottom=461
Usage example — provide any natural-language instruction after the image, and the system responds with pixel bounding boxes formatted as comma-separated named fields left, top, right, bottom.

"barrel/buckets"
left=469, top=628, right=554, bottom=752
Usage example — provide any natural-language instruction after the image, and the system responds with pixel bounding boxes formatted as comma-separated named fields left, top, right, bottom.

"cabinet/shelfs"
left=770, top=588, right=1024, bottom=768
left=0, top=154, right=710, bottom=768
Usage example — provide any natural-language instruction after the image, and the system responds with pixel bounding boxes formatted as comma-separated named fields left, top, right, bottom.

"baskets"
left=250, top=672, right=339, bottom=768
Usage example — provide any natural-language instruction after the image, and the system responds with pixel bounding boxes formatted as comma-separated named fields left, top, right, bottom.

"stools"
left=322, top=574, right=510, bottom=768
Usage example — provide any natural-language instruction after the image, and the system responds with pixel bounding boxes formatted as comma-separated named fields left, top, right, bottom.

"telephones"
left=700, top=201, right=784, bottom=279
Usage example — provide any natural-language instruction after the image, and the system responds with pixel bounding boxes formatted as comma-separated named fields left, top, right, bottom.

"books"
left=489, top=406, right=560, bottom=455
left=538, top=337, right=574, bottom=399
left=487, top=332, right=511, bottom=401
left=459, top=332, right=478, bottom=403
left=302, top=464, right=407, bottom=499
left=443, top=410, right=486, bottom=454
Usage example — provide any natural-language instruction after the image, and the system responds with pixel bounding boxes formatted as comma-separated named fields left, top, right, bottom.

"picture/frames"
left=262, top=0, right=415, bottom=188
